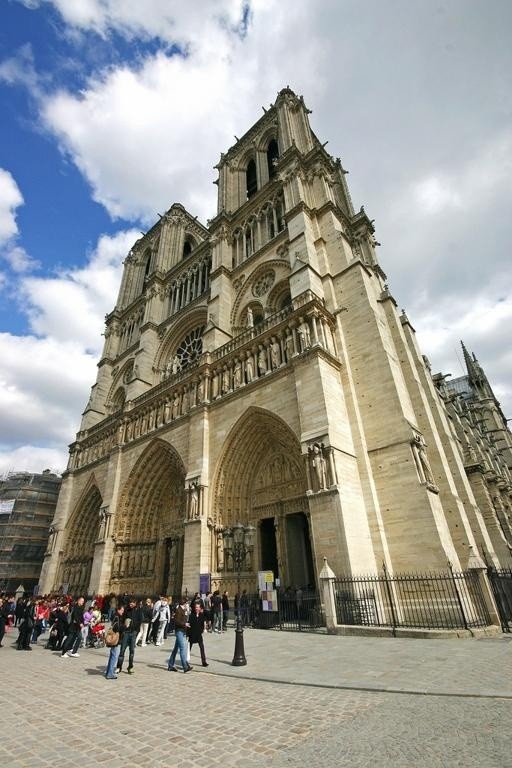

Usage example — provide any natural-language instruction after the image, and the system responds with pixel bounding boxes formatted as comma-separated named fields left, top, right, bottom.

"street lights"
left=221, top=521, right=257, bottom=664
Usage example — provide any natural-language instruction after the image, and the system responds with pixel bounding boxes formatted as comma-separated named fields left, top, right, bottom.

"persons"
left=74, top=306, right=311, bottom=467
left=312, top=446, right=329, bottom=490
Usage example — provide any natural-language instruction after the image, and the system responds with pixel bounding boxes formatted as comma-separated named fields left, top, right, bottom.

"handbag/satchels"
left=105, top=628, right=119, bottom=646
left=37, top=631, right=50, bottom=645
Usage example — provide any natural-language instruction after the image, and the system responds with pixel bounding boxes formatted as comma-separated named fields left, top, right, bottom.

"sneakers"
left=140, top=639, right=167, bottom=647
left=106, top=667, right=134, bottom=679
left=184, top=667, right=193, bottom=673
left=71, top=653, right=80, bottom=657
left=61, top=653, right=69, bottom=658
left=172, top=668, right=177, bottom=671
left=204, top=663, right=208, bottom=667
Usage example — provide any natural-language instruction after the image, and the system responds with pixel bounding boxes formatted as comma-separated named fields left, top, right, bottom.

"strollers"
left=89, top=619, right=106, bottom=648
